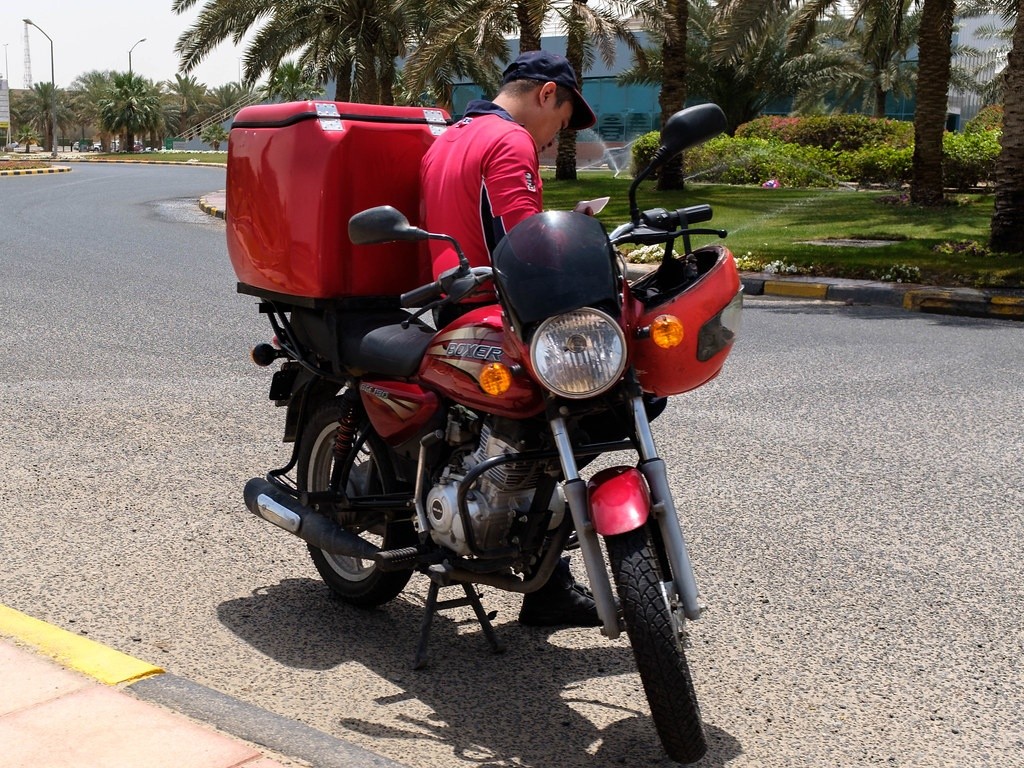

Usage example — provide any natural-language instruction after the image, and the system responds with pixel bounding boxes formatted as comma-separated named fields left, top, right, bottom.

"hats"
left=504, top=51, right=597, bottom=133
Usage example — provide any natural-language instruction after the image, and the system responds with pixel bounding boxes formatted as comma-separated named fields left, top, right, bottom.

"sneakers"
left=519, top=557, right=624, bottom=628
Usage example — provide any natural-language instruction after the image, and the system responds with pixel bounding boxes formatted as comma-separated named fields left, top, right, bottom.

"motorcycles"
left=225, top=100, right=744, bottom=765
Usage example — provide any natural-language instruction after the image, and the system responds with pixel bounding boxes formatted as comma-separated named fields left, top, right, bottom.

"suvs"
left=602, top=139, right=636, bottom=170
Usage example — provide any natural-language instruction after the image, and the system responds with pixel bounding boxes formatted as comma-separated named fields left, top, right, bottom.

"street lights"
left=237, top=55, right=241, bottom=82
left=23, top=18, right=58, bottom=157
left=129, top=38, right=146, bottom=73
left=3, top=43, right=10, bottom=80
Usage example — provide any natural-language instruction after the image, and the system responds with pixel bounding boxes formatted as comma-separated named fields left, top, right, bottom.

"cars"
left=74, top=140, right=158, bottom=153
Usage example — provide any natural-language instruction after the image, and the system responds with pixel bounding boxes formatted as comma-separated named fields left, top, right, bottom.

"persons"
left=419, top=52, right=622, bottom=627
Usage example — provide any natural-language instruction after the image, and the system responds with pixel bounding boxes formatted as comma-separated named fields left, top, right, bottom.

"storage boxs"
left=224, top=100, right=454, bottom=299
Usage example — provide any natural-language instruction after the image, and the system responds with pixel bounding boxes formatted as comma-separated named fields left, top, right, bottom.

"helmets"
left=620, top=244, right=744, bottom=395
